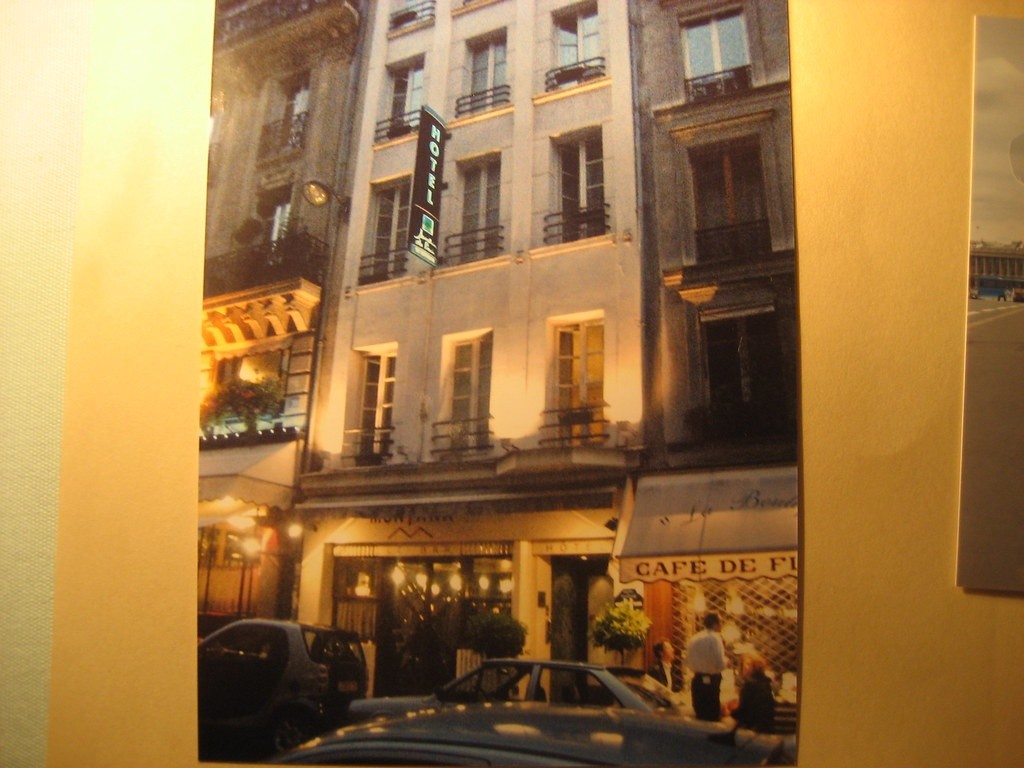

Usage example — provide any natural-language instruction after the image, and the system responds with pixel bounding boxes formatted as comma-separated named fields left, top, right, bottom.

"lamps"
left=301, top=180, right=353, bottom=223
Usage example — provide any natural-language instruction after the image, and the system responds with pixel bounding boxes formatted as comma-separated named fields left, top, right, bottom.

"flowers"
left=199, top=375, right=281, bottom=428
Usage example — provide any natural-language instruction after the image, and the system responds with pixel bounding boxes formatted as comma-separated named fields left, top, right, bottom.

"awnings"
left=618, top=463, right=798, bottom=584
left=198, top=438, right=297, bottom=512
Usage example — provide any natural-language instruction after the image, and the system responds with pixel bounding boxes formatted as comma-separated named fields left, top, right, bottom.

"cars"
left=197, top=618, right=366, bottom=759
left=274, top=702, right=799, bottom=768
left=1012, top=288, right=1024, bottom=302
left=349, top=657, right=695, bottom=718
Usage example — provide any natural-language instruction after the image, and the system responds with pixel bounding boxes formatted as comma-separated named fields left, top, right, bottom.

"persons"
left=685, top=610, right=730, bottom=722
left=648, top=641, right=685, bottom=692
left=727, top=656, right=774, bottom=734
